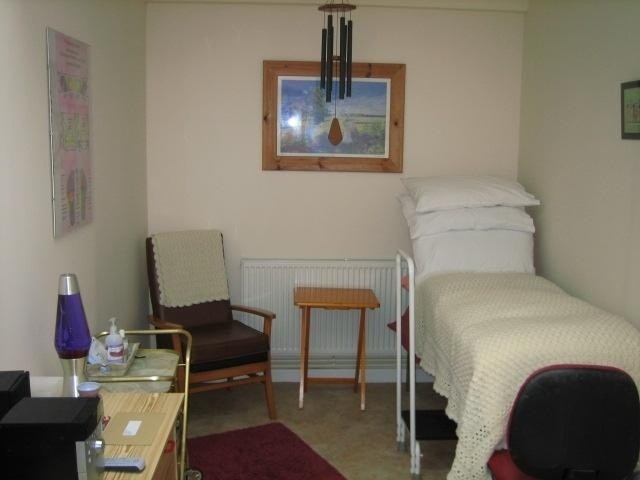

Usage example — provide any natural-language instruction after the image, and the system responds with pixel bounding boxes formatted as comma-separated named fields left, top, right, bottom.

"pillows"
left=399, top=172, right=541, bottom=215
left=398, top=190, right=539, bottom=238
left=408, top=229, right=539, bottom=279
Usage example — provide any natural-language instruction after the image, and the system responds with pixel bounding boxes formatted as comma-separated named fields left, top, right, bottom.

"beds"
left=402, top=275, right=640, bottom=480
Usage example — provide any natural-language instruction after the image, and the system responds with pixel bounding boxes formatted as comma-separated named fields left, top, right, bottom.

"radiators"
left=240, top=256, right=414, bottom=357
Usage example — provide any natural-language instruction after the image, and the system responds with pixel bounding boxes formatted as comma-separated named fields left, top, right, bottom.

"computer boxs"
left=0, top=398, right=104, bottom=480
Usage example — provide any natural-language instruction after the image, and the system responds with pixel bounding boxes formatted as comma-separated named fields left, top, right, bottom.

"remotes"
left=104, top=457, right=145, bottom=472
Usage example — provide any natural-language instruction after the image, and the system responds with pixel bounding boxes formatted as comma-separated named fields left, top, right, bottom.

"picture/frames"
left=620, top=79, right=640, bottom=140
left=261, top=59, right=408, bottom=175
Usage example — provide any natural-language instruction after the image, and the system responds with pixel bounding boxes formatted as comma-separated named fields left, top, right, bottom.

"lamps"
left=47, top=268, right=103, bottom=398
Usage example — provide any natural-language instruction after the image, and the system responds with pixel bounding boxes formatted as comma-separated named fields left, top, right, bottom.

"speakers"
left=0, top=371, right=30, bottom=420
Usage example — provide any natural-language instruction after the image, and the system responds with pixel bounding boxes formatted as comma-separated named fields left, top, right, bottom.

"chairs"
left=507, top=361, right=640, bottom=479
left=138, top=227, right=280, bottom=420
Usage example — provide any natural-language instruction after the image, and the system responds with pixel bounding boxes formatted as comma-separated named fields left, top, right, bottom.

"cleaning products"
left=104, top=317, right=124, bottom=364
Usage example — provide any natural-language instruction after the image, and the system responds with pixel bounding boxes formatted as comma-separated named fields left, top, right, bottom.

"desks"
left=288, top=286, right=378, bottom=412
left=484, top=448, right=520, bottom=479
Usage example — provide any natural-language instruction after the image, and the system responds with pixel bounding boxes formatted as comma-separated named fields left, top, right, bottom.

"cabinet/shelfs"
left=84, top=328, right=201, bottom=477
left=1, top=390, right=190, bottom=479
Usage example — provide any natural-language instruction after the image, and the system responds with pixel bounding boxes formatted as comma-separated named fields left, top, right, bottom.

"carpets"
left=185, top=418, right=347, bottom=479
